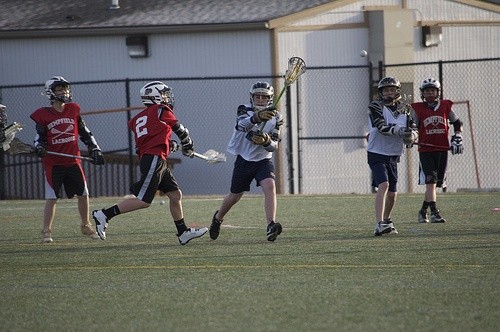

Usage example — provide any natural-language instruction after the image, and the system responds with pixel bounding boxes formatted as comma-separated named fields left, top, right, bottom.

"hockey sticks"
left=397, top=93, right=413, bottom=149
left=366, top=132, right=464, bottom=150
left=253, top=55, right=307, bottom=145
left=178, top=145, right=227, bottom=166
left=6, top=136, right=93, bottom=161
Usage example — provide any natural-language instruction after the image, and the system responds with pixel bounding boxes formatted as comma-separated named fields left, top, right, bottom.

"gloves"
left=448, top=134, right=463, bottom=155
left=252, top=130, right=271, bottom=147
left=253, top=107, right=276, bottom=124
left=182, top=146, right=194, bottom=158
left=90, top=150, right=106, bottom=165
left=399, top=126, right=415, bottom=145
left=36, top=145, right=47, bottom=158
left=169, top=140, right=178, bottom=152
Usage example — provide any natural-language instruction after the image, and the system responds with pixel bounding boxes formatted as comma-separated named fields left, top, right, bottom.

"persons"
left=0, top=105, right=22, bottom=152
left=29, top=75, right=103, bottom=241
left=93, top=81, right=209, bottom=245
left=366, top=76, right=419, bottom=236
left=209, top=81, right=283, bottom=241
left=410, top=78, right=464, bottom=222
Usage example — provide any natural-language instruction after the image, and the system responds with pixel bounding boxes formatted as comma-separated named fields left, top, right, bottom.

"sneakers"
left=178, top=227, right=207, bottom=245
left=386, top=222, right=398, bottom=234
left=92, top=210, right=108, bottom=240
left=431, top=213, right=446, bottom=223
left=209, top=210, right=223, bottom=240
left=266, top=216, right=282, bottom=241
left=374, top=220, right=392, bottom=236
left=417, top=211, right=430, bottom=223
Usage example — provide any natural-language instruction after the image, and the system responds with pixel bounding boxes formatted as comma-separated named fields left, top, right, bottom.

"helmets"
left=45, top=77, right=72, bottom=105
left=140, top=81, right=174, bottom=108
left=378, top=76, right=400, bottom=104
left=421, top=78, right=440, bottom=95
left=249, top=82, right=274, bottom=110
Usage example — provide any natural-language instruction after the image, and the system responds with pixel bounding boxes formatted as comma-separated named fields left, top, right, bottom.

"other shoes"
left=81, top=223, right=99, bottom=238
left=40, top=228, right=53, bottom=242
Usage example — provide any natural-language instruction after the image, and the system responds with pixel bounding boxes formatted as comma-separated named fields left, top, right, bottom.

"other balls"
left=360, top=50, right=367, bottom=57
